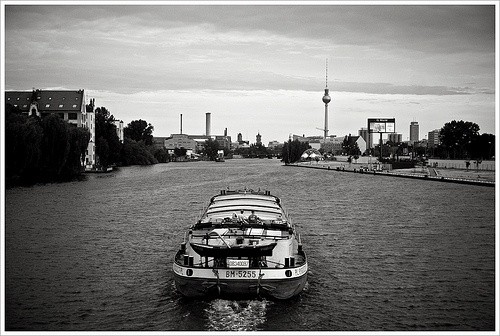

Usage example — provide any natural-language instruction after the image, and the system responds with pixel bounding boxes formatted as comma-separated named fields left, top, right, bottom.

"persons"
left=247, top=210, right=258, bottom=224
left=237, top=210, right=247, bottom=224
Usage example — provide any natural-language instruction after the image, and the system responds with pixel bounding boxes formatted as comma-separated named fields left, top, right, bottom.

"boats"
left=83, top=164, right=114, bottom=173
left=215, top=150, right=225, bottom=163
left=171, top=185, right=309, bottom=301
